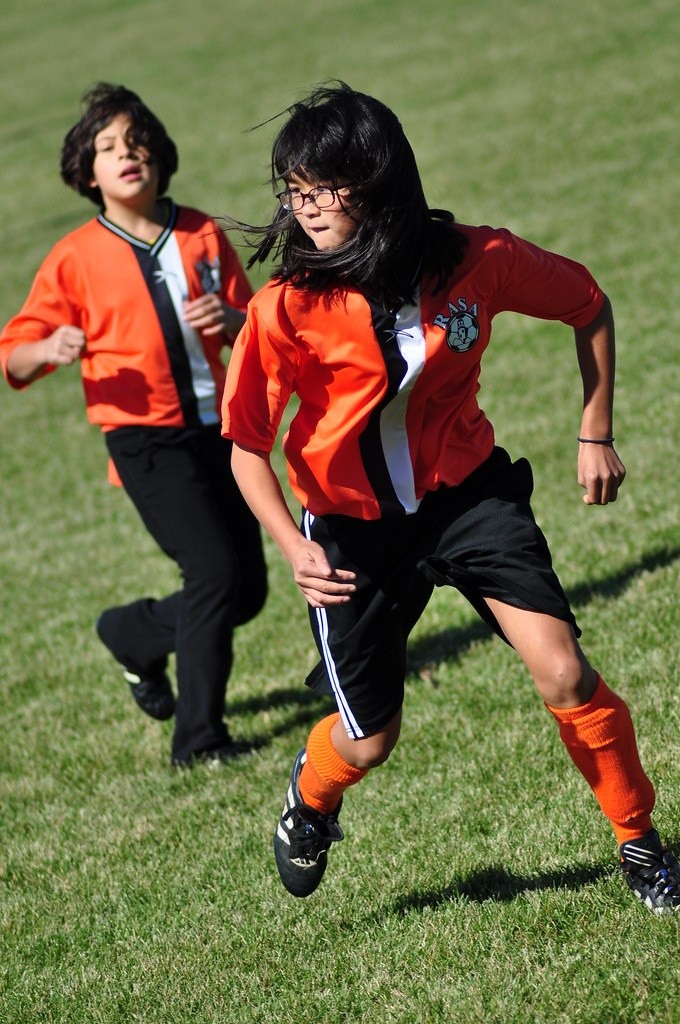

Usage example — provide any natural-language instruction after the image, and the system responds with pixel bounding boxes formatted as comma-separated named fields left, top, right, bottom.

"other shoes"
left=98, top=608, right=175, bottom=720
left=169, top=743, right=251, bottom=770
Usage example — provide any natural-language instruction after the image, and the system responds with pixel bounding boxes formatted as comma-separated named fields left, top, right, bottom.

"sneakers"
left=619, top=827, right=680, bottom=916
left=271, top=749, right=343, bottom=898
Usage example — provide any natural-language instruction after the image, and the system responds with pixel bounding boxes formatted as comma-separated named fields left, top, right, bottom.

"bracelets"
left=577, top=438, right=614, bottom=442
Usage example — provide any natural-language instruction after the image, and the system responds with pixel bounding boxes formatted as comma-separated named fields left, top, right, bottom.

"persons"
left=0, top=88, right=268, bottom=771
left=221, top=86, right=680, bottom=917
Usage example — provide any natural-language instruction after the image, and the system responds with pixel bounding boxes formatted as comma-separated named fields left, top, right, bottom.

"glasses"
left=275, top=181, right=356, bottom=211
